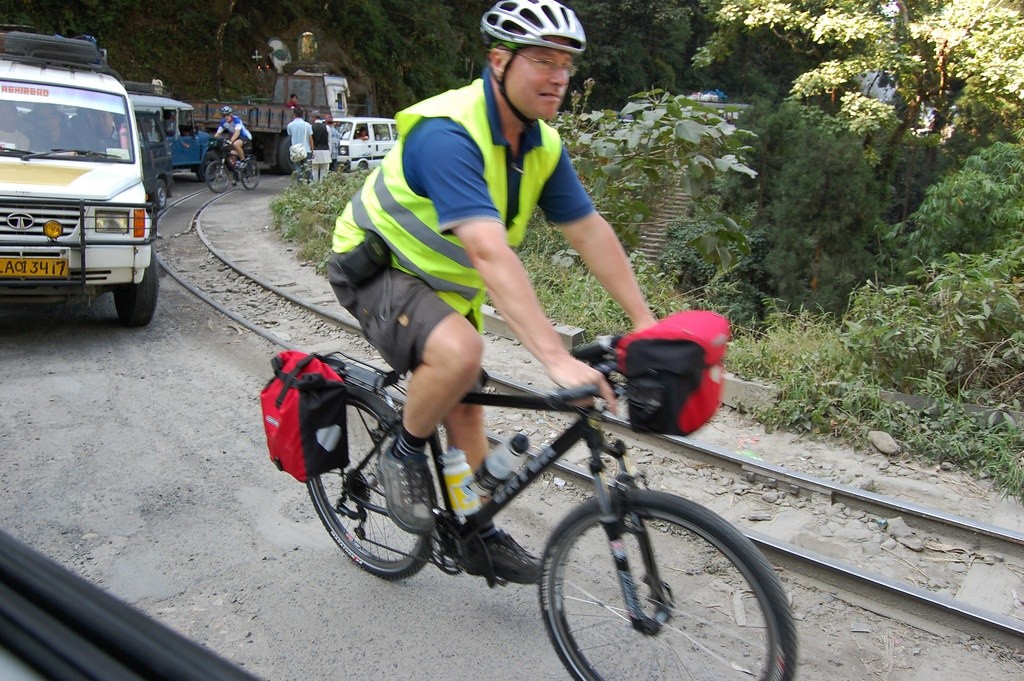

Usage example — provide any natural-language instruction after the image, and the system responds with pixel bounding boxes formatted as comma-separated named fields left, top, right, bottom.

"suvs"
left=0, top=33, right=159, bottom=331
left=128, top=92, right=217, bottom=182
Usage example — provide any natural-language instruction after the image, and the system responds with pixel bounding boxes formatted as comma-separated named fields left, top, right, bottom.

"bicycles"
left=307, top=332, right=798, bottom=681
left=206, top=137, right=261, bottom=194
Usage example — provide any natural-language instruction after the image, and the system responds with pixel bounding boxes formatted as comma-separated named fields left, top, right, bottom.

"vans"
left=335, top=117, right=397, bottom=173
left=136, top=112, right=174, bottom=209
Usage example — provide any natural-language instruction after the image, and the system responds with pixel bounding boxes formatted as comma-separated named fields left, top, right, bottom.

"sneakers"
left=461, top=530, right=544, bottom=583
left=377, top=437, right=433, bottom=532
left=238, top=161, right=247, bottom=169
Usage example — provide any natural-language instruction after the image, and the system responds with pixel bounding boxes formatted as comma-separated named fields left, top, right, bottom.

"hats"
left=311, top=110, right=321, bottom=116
left=325, top=115, right=333, bottom=122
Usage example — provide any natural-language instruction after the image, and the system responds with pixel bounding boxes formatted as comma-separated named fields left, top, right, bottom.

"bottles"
left=441, top=448, right=482, bottom=519
left=229, top=158, right=235, bottom=166
left=472, top=433, right=530, bottom=497
left=236, top=160, right=240, bottom=168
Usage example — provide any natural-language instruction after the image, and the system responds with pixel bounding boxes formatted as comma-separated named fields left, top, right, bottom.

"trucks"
left=185, top=72, right=354, bottom=173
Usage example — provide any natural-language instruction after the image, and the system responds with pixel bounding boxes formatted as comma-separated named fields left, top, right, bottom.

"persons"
left=161, top=111, right=180, bottom=137
left=311, top=109, right=330, bottom=185
left=0, top=101, right=121, bottom=154
left=327, top=0, right=661, bottom=585
left=286, top=108, right=315, bottom=188
left=375, top=133, right=381, bottom=140
left=354, top=126, right=368, bottom=140
left=285, top=93, right=304, bottom=111
left=139, top=121, right=159, bottom=142
left=324, top=114, right=340, bottom=173
left=208, top=106, right=252, bottom=185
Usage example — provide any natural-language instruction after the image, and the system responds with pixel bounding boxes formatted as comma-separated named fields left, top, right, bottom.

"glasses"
left=223, top=114, right=230, bottom=117
left=486, top=47, right=580, bottom=77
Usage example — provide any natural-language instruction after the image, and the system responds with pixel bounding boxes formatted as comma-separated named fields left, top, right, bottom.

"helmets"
left=479, top=0, right=587, bottom=55
left=220, top=105, right=233, bottom=114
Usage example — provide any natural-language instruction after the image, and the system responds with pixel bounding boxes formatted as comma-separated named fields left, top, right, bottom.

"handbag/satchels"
left=289, top=143, right=307, bottom=162
left=613, top=308, right=732, bottom=437
left=259, top=348, right=348, bottom=483
